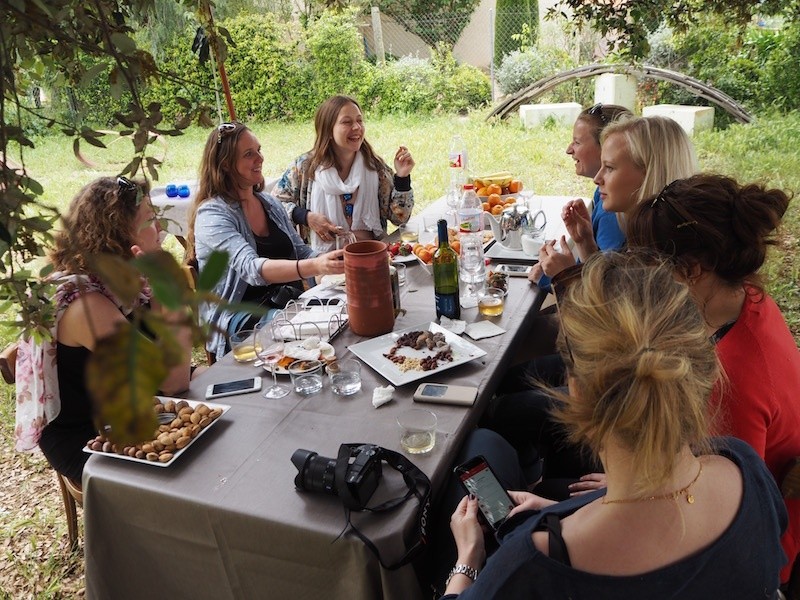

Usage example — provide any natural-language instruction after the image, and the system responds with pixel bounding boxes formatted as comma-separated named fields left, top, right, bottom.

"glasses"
left=550, top=249, right=662, bottom=366
left=590, top=102, right=607, bottom=125
left=215, top=122, right=243, bottom=157
left=651, top=179, right=705, bottom=242
left=117, top=176, right=138, bottom=195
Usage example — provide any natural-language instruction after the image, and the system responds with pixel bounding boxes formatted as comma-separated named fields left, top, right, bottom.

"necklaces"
left=601, top=460, right=704, bottom=504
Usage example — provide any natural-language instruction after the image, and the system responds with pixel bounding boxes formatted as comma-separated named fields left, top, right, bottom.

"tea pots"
left=483, top=204, right=547, bottom=251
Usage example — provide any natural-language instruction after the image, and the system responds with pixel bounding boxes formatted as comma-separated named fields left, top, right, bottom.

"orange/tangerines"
left=414, top=241, right=460, bottom=262
left=472, top=179, right=523, bottom=215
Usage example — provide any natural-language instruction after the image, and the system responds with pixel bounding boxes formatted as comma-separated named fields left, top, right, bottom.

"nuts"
left=86, top=397, right=223, bottom=463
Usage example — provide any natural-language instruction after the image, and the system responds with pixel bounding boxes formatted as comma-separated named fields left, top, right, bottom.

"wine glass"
left=458, top=234, right=485, bottom=305
left=253, top=321, right=291, bottom=399
left=517, top=173, right=534, bottom=207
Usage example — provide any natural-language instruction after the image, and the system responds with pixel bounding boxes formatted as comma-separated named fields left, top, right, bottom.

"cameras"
left=289, top=442, right=383, bottom=513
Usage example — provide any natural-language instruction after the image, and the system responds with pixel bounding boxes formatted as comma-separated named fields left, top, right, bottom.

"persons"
left=440, top=103, right=800, bottom=600
left=14, top=96, right=415, bottom=479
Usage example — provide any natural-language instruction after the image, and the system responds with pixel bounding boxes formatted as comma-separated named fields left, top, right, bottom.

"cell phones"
left=412, top=383, right=478, bottom=406
left=494, top=263, right=532, bottom=277
left=453, top=455, right=516, bottom=533
left=204, top=376, right=262, bottom=399
left=305, top=298, right=341, bottom=307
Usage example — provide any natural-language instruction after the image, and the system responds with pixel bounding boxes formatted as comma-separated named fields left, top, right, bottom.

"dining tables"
left=83, top=193, right=633, bottom=600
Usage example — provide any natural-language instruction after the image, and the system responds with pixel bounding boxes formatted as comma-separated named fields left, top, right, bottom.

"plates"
left=390, top=242, right=417, bottom=263
left=262, top=339, right=336, bottom=375
left=484, top=240, right=563, bottom=260
left=82, top=395, right=232, bottom=467
left=347, top=321, right=489, bottom=387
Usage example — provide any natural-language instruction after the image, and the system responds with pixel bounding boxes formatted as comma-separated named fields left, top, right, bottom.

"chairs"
left=0, top=343, right=83, bottom=551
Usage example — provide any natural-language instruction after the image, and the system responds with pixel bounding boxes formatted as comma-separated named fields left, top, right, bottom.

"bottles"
left=432, top=218, right=461, bottom=320
left=459, top=184, right=485, bottom=284
left=449, top=136, right=468, bottom=211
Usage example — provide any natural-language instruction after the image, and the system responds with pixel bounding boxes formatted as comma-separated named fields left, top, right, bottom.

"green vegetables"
left=397, top=239, right=413, bottom=256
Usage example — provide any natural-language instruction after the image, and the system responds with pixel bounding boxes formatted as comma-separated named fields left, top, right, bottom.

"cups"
left=389, top=264, right=400, bottom=319
left=396, top=409, right=438, bottom=455
left=343, top=240, right=395, bottom=338
left=287, top=359, right=323, bottom=396
left=484, top=271, right=511, bottom=298
left=423, top=214, right=442, bottom=232
left=229, top=329, right=264, bottom=363
left=398, top=223, right=420, bottom=247
left=476, top=287, right=504, bottom=319
left=326, top=359, right=362, bottom=396
left=165, top=184, right=190, bottom=198
left=335, top=232, right=358, bottom=261
left=391, top=263, right=406, bottom=288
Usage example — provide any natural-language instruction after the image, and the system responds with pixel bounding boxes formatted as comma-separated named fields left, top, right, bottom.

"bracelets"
left=297, top=259, right=307, bottom=280
left=445, top=564, right=478, bottom=586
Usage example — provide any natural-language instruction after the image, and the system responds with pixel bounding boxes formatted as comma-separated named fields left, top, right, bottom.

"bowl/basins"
left=411, top=250, right=461, bottom=276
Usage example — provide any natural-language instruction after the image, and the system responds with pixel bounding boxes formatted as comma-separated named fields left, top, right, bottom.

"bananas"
left=481, top=171, right=512, bottom=188
